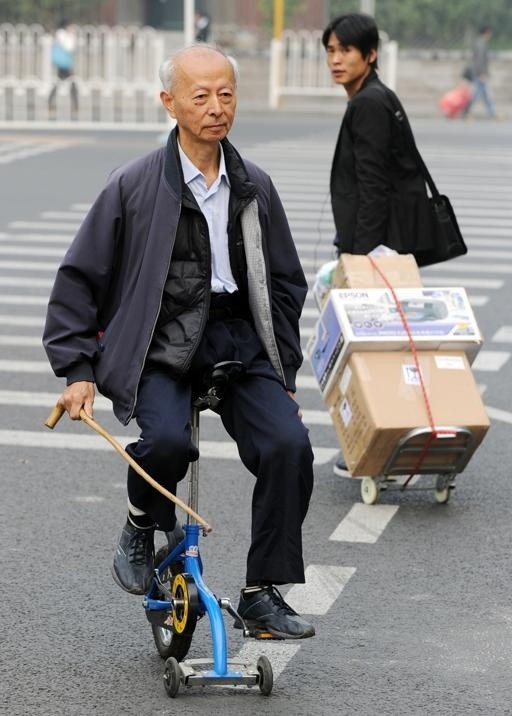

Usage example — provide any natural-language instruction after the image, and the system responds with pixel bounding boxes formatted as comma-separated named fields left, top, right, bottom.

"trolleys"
left=307, top=251, right=477, bottom=509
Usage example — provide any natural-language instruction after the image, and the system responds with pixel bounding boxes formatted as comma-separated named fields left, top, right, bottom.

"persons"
left=42, top=45, right=314, bottom=639
left=47, top=17, right=78, bottom=112
left=194, top=9, right=210, bottom=43
left=461, top=27, right=505, bottom=123
left=323, top=14, right=431, bottom=481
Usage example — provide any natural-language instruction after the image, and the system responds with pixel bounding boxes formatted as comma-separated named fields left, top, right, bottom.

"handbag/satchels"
left=465, top=66, right=474, bottom=79
left=415, top=195, right=468, bottom=268
left=51, top=42, right=74, bottom=70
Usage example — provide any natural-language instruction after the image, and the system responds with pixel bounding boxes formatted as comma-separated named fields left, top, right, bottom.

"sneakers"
left=235, top=585, right=316, bottom=638
left=111, top=524, right=155, bottom=595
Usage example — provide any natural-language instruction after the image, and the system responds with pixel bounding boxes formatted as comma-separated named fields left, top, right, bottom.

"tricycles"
left=138, top=348, right=277, bottom=699
